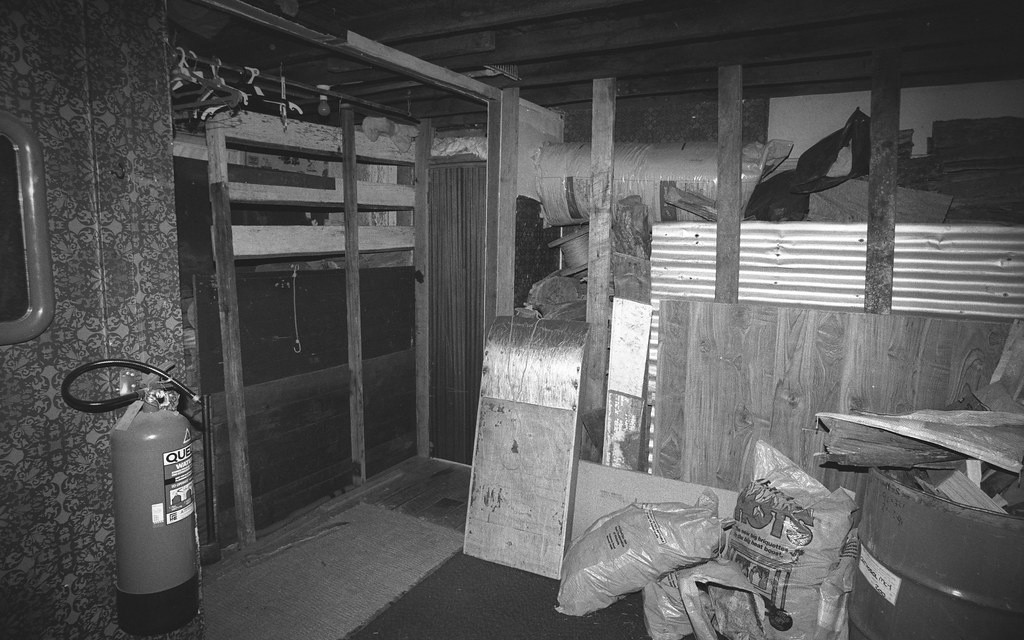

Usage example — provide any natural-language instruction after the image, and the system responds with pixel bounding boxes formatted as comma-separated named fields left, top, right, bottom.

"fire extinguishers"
left=61, top=359, right=206, bottom=637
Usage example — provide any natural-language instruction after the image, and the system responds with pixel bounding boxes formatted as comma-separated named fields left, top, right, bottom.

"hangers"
left=171, top=44, right=305, bottom=121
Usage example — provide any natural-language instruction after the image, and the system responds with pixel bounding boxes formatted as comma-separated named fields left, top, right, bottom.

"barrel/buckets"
left=847, top=467, right=1024, bottom=640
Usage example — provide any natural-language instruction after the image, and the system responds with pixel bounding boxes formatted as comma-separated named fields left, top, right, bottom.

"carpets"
left=202, top=499, right=465, bottom=640
